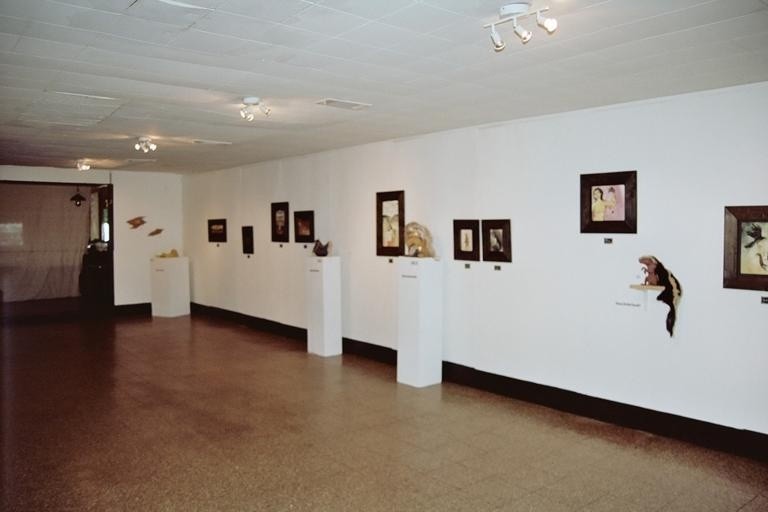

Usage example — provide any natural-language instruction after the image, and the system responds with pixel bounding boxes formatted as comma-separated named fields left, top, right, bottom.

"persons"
left=591, top=187, right=616, bottom=222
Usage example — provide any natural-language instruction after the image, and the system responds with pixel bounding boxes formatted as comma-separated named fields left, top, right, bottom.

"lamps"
left=238, top=96, right=272, bottom=122
left=132, top=135, right=157, bottom=154
left=70, top=185, right=87, bottom=207
left=482, top=1, right=559, bottom=52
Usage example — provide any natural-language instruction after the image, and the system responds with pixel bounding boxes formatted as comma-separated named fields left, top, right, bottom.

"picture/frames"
left=241, top=226, right=253, bottom=254
left=722, top=204, right=768, bottom=292
left=579, top=170, right=637, bottom=234
left=293, top=210, right=314, bottom=243
left=375, top=189, right=405, bottom=256
left=452, top=219, right=479, bottom=261
left=481, top=218, right=512, bottom=263
left=270, top=201, right=289, bottom=243
left=207, top=218, right=226, bottom=243
